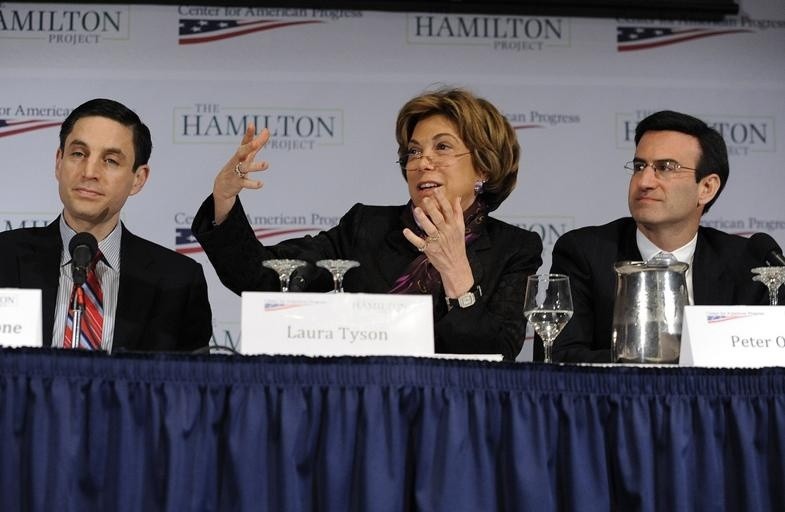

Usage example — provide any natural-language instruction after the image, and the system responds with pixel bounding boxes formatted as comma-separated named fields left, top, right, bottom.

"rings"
left=428, top=233, right=440, bottom=241
left=235, top=162, right=247, bottom=177
left=418, top=241, right=428, bottom=251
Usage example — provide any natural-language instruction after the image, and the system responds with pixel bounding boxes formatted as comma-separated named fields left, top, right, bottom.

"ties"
left=62, top=250, right=105, bottom=350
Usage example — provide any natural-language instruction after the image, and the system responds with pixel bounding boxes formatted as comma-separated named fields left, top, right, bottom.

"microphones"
left=68, top=231, right=99, bottom=286
left=745, top=232, right=785, bottom=266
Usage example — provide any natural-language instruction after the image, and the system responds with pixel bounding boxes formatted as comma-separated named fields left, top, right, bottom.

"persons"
left=191, top=77, right=544, bottom=361
left=533, top=110, right=785, bottom=366
left=1, top=97, right=214, bottom=351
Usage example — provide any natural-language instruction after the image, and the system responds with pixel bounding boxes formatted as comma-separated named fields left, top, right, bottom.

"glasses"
left=393, top=149, right=477, bottom=173
left=621, top=160, right=701, bottom=176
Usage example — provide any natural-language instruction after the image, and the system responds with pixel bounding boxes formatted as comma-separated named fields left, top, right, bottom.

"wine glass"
left=750, top=265, right=785, bottom=304
left=313, top=255, right=360, bottom=294
left=261, top=252, right=305, bottom=292
left=519, top=270, right=577, bottom=363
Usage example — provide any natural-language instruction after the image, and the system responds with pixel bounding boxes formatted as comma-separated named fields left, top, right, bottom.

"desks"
left=1, top=351, right=784, bottom=511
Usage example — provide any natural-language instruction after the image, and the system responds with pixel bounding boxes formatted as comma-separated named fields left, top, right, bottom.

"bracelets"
left=445, top=283, right=483, bottom=308
